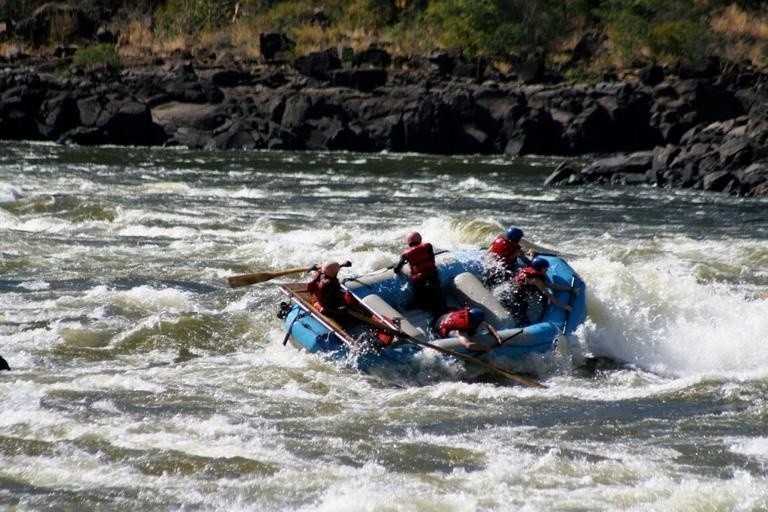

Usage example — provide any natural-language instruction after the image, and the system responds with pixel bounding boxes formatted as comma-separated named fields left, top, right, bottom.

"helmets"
left=321, top=261, right=340, bottom=278
left=407, top=231, right=421, bottom=244
left=531, top=258, right=549, bottom=272
left=469, top=308, right=485, bottom=328
left=506, top=227, right=523, bottom=241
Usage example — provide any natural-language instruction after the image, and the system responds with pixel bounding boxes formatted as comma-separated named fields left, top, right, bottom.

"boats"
left=285, top=252, right=585, bottom=375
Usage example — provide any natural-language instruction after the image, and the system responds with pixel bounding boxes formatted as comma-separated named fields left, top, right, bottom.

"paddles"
left=364, top=251, right=448, bottom=275
left=558, top=274, right=575, bottom=352
left=347, top=310, right=547, bottom=389
left=227, top=261, right=351, bottom=290
left=458, top=244, right=577, bottom=260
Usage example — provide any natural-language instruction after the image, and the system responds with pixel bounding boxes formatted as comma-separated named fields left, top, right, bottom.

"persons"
left=483, top=224, right=538, bottom=295
left=494, top=257, right=580, bottom=328
left=306, top=260, right=402, bottom=335
left=428, top=304, right=502, bottom=352
left=392, top=230, right=449, bottom=319
